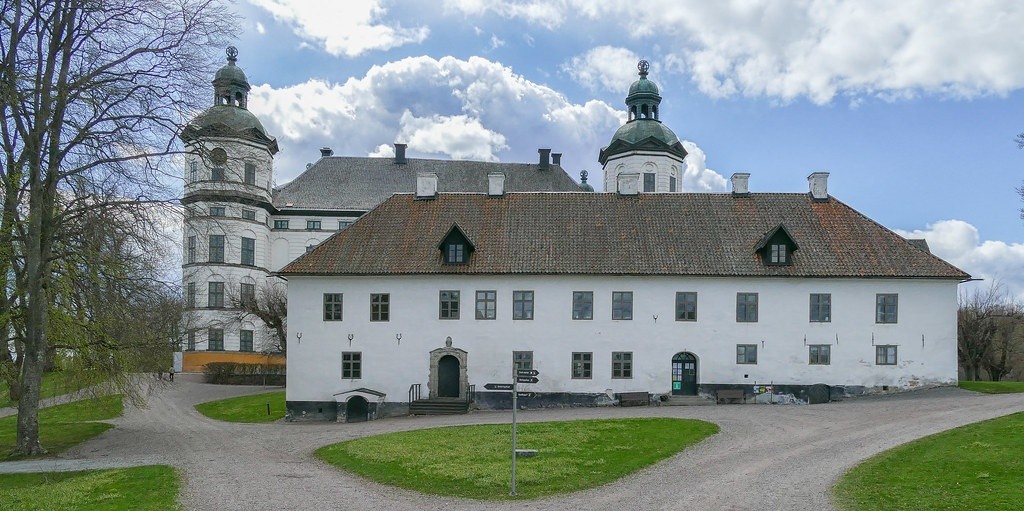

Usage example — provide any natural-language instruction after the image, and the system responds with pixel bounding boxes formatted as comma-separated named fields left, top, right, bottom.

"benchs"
left=715, top=389, right=746, bottom=405
left=620, top=392, right=650, bottom=406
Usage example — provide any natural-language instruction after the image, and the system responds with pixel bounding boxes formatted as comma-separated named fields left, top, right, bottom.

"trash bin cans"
left=798, top=383, right=846, bottom=404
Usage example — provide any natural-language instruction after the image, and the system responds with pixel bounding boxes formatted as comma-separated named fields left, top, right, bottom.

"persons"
left=169, top=366, right=175, bottom=382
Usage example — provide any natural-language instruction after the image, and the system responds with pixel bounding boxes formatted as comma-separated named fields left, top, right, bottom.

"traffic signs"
left=482, top=368, right=540, bottom=399
